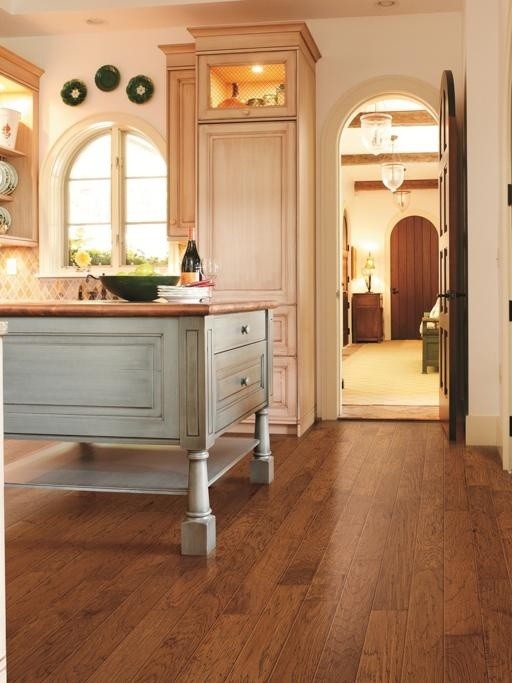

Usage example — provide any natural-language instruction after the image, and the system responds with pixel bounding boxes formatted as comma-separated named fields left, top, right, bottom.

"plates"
left=156, top=284, right=210, bottom=305
left=0, top=159, right=20, bottom=233
left=60, top=78, right=87, bottom=106
left=95, top=65, right=121, bottom=91
left=127, top=74, right=153, bottom=103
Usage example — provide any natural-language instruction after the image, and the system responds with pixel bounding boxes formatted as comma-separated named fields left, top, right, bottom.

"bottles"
left=180, top=227, right=203, bottom=284
left=246, top=83, right=287, bottom=107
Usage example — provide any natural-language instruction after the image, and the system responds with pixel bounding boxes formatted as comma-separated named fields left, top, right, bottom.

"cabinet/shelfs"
left=0, top=308, right=274, bottom=556
left=0, top=44, right=44, bottom=248
left=350, top=293, right=384, bottom=343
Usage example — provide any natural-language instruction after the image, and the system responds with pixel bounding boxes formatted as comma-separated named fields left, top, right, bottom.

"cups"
left=0, top=106, right=21, bottom=150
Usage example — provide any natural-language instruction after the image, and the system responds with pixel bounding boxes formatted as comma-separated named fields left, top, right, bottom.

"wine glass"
left=200, top=256, right=222, bottom=304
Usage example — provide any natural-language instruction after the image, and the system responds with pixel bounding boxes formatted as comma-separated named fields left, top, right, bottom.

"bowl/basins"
left=98, top=274, right=182, bottom=302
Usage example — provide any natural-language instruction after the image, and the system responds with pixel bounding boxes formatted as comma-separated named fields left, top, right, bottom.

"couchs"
left=418, top=312, right=440, bottom=374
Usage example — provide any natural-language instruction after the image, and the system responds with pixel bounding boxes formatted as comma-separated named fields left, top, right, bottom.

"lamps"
left=359, top=101, right=412, bottom=212
left=363, top=251, right=375, bottom=293
